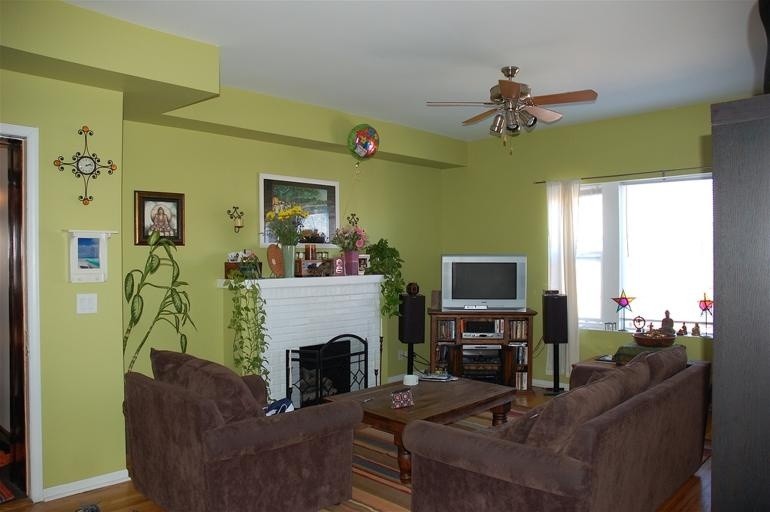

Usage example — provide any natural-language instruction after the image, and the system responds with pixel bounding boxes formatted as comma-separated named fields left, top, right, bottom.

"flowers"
left=265, top=202, right=307, bottom=246
left=332, top=222, right=368, bottom=251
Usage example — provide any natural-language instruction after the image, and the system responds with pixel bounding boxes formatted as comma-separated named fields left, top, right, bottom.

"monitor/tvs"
left=442, top=255, right=528, bottom=312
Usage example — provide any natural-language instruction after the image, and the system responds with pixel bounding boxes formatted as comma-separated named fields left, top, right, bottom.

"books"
left=435, top=319, right=529, bottom=391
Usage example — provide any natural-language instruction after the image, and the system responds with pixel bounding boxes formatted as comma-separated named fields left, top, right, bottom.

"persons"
left=153, top=207, right=168, bottom=228
left=662, top=310, right=673, bottom=328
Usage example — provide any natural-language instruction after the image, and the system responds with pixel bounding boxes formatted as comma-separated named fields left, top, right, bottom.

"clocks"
left=54, top=124, right=117, bottom=206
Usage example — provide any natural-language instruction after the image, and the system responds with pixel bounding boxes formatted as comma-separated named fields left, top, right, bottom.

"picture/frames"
left=134, top=189, right=187, bottom=247
left=255, top=170, right=339, bottom=249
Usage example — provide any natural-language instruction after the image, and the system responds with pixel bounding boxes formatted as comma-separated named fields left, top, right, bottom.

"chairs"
left=123, top=348, right=365, bottom=511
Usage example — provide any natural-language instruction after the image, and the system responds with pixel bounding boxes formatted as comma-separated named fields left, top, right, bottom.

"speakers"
left=398, top=294, right=426, bottom=344
left=543, top=294, right=569, bottom=344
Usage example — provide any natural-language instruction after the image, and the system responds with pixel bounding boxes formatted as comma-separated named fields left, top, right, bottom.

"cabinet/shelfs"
left=711, top=93, right=770, bottom=512
left=427, top=300, right=540, bottom=395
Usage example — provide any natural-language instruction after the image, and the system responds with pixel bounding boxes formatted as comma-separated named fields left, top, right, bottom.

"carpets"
left=320, top=405, right=533, bottom=512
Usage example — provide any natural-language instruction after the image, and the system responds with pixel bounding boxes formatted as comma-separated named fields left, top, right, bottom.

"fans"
left=427, top=66, right=599, bottom=125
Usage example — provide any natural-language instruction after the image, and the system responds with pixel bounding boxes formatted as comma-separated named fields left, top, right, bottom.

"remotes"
left=422, top=374, right=449, bottom=380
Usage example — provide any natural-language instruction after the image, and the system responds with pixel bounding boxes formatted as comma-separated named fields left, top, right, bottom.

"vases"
left=281, top=244, right=295, bottom=278
left=344, top=250, right=359, bottom=275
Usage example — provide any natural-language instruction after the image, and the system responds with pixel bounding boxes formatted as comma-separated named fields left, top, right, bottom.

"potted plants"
left=224, top=253, right=276, bottom=402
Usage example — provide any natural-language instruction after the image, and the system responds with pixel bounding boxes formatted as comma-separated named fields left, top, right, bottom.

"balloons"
left=347, top=123, right=379, bottom=161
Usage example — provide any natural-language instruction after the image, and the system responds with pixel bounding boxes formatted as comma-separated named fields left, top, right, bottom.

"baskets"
left=632, top=333, right=677, bottom=347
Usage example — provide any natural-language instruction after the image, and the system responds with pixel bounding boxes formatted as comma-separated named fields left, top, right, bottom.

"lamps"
left=490, top=101, right=537, bottom=134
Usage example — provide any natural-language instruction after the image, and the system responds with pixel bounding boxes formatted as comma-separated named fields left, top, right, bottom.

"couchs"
left=400, top=345, right=713, bottom=510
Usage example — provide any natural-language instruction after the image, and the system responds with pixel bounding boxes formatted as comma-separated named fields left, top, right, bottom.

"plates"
left=267, top=244, right=283, bottom=277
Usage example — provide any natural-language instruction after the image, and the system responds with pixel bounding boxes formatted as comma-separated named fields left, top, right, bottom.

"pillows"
left=150, top=349, right=265, bottom=422
left=502, top=347, right=686, bottom=451
